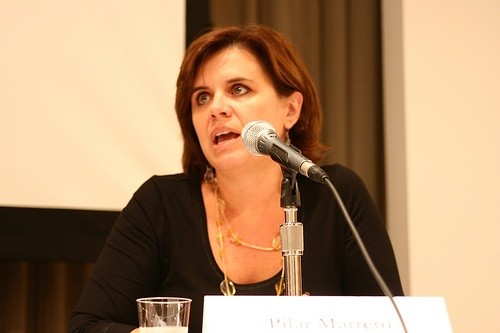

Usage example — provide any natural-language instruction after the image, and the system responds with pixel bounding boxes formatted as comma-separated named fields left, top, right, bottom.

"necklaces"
left=202, top=164, right=284, bottom=296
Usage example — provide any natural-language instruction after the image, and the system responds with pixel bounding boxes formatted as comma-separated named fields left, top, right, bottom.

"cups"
left=136, top=296, right=192, bottom=333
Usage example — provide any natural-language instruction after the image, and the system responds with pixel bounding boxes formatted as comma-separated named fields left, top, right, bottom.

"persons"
left=67, top=22, right=405, bottom=333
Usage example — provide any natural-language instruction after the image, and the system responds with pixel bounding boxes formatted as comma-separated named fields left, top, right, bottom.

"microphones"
left=242, top=120, right=329, bottom=184
